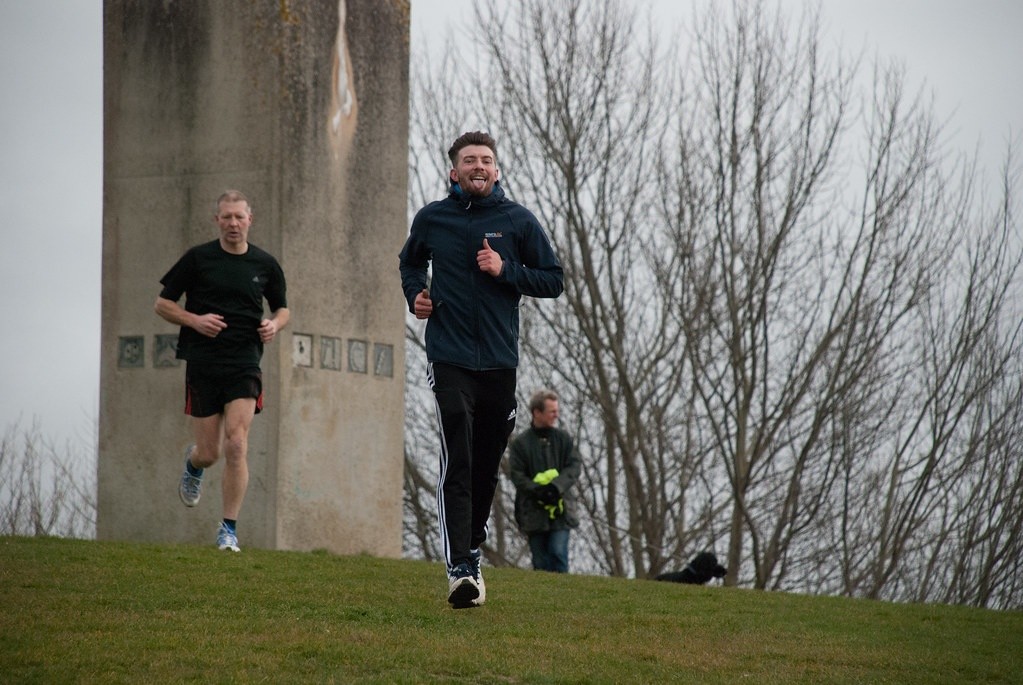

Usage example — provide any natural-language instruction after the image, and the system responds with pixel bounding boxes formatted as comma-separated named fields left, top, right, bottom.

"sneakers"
left=216, top=522, right=241, bottom=552
left=179, top=445, right=204, bottom=508
left=447, top=549, right=486, bottom=608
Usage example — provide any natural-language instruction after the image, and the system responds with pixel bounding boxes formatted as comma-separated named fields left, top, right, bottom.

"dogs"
left=655, top=551, right=728, bottom=585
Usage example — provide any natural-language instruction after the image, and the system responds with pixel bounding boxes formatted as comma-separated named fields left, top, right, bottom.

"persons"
left=154, top=191, right=290, bottom=552
left=398, top=132, right=564, bottom=608
left=509, top=392, right=582, bottom=572
